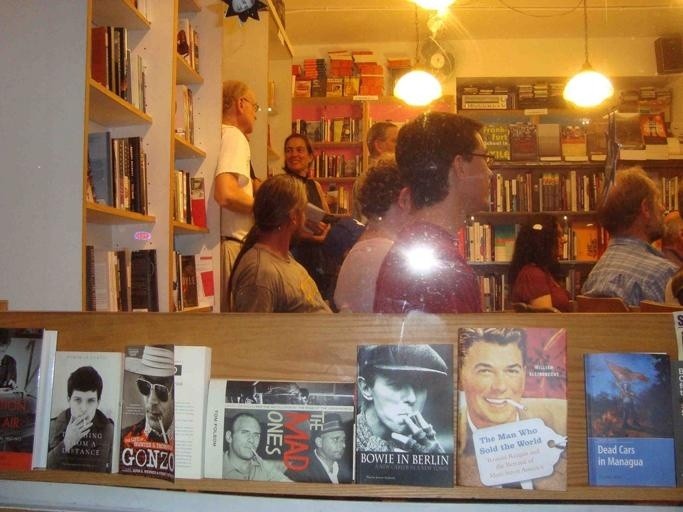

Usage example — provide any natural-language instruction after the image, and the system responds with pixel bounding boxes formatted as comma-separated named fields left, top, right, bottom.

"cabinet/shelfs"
left=451, top=74, right=683, bottom=312
left=171, top=1, right=221, bottom=313
left=224, top=1, right=294, bottom=179
left=292, top=98, right=366, bottom=217
left=1, top=1, right=176, bottom=312
left=366, top=95, right=454, bottom=162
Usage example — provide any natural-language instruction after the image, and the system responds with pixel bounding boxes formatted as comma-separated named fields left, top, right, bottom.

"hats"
left=124, top=346, right=177, bottom=376
left=361, top=344, right=448, bottom=377
left=314, top=414, right=345, bottom=438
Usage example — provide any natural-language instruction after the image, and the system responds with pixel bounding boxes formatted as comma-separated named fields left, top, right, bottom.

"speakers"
left=655, top=36, right=682, bottom=75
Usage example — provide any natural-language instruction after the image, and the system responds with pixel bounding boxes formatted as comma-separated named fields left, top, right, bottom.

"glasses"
left=243, top=98, right=259, bottom=112
left=464, top=152, right=495, bottom=166
left=136, top=375, right=174, bottom=401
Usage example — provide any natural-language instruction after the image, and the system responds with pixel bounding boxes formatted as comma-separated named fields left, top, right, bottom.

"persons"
left=663, top=210, right=683, bottom=265
left=356, top=345, right=448, bottom=453
left=511, top=212, right=571, bottom=313
left=0, top=328, right=16, bottom=388
left=615, top=382, right=645, bottom=428
left=47, top=367, right=114, bottom=472
left=583, top=166, right=681, bottom=306
left=216, top=79, right=262, bottom=313
left=326, top=214, right=365, bottom=252
left=458, top=327, right=567, bottom=490
left=335, top=158, right=414, bottom=314
left=368, top=122, right=396, bottom=157
left=120, top=346, right=175, bottom=483
left=222, top=413, right=294, bottom=481
left=228, top=175, right=333, bottom=315
left=265, top=382, right=307, bottom=400
left=283, top=134, right=330, bottom=297
left=374, top=111, right=496, bottom=314
left=283, top=414, right=352, bottom=484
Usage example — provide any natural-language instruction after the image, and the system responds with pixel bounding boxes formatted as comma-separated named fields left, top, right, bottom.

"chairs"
left=575, top=294, right=630, bottom=313
left=509, top=302, right=526, bottom=313
left=639, top=299, right=683, bottom=313
left=527, top=305, right=560, bottom=313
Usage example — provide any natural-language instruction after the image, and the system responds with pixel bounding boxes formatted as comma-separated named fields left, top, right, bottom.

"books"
left=46, top=352, right=126, bottom=473
left=291, top=48, right=412, bottom=102
left=306, top=149, right=364, bottom=178
left=87, top=131, right=149, bottom=216
left=356, top=343, right=455, bottom=486
left=478, top=116, right=683, bottom=164
left=583, top=353, right=678, bottom=489
left=320, top=182, right=354, bottom=213
left=0, top=328, right=58, bottom=470
left=488, top=167, right=683, bottom=213
left=84, top=243, right=157, bottom=312
left=459, top=216, right=664, bottom=261
left=174, top=84, right=197, bottom=143
left=172, top=250, right=216, bottom=309
left=205, top=378, right=356, bottom=485
left=178, top=17, right=203, bottom=76
left=173, top=169, right=208, bottom=229
left=120, top=344, right=211, bottom=478
left=268, top=77, right=278, bottom=110
left=473, top=264, right=584, bottom=312
left=125, top=0, right=152, bottom=23
left=291, top=115, right=367, bottom=144
left=458, top=81, right=670, bottom=112
left=90, top=27, right=149, bottom=117
left=457, top=326, right=568, bottom=489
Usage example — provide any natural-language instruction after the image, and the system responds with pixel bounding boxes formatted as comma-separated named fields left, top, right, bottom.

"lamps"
left=393, top=0, right=443, bottom=107
left=562, top=0, right=615, bottom=109
